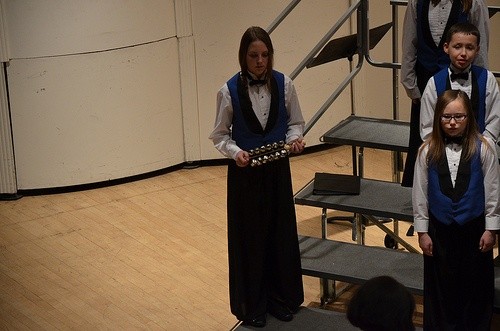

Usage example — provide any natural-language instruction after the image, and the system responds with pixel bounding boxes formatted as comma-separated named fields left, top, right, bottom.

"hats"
left=347, top=276, right=415, bottom=331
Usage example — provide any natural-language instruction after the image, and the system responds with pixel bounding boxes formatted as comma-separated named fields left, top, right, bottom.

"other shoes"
left=251, top=317, right=266, bottom=328
left=271, top=310, right=293, bottom=321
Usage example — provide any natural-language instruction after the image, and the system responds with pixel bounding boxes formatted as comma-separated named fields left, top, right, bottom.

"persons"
left=347, top=276, right=417, bottom=331
left=209, top=26, right=307, bottom=327
left=400, top=0, right=490, bottom=188
left=412, top=89, right=500, bottom=331
left=420, top=22, right=500, bottom=146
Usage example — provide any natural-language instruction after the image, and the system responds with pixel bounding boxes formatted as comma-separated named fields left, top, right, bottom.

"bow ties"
left=246, top=72, right=268, bottom=86
left=450, top=67, right=471, bottom=80
left=445, top=135, right=464, bottom=146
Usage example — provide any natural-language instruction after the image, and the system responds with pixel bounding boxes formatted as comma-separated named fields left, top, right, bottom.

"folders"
left=313, top=172, right=360, bottom=195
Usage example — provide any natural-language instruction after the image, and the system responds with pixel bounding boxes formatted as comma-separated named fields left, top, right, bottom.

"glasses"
left=440, top=114, right=468, bottom=123
